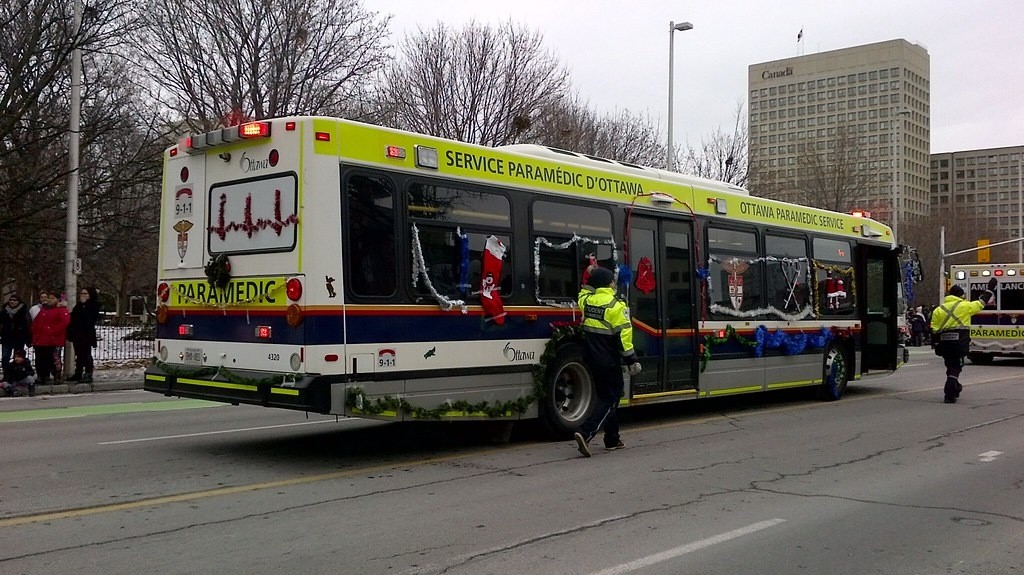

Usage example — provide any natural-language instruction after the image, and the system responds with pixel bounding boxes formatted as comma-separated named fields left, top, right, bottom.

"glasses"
left=81, top=293, right=89, bottom=295
left=9, top=299, right=18, bottom=301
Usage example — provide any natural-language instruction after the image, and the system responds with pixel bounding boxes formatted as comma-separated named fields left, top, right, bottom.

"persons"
left=2, top=350, right=35, bottom=397
left=907, top=303, right=939, bottom=346
left=0, top=294, right=32, bottom=369
left=31, top=292, right=71, bottom=385
left=928, top=278, right=997, bottom=402
left=65, top=287, right=99, bottom=379
left=28, top=291, right=49, bottom=320
left=574, top=268, right=642, bottom=456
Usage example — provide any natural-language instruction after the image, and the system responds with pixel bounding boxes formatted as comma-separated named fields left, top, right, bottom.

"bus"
left=144, top=115, right=924, bottom=437
left=948, top=264, right=1024, bottom=363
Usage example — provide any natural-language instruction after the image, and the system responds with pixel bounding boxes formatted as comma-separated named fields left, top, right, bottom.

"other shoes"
left=604, top=439, right=625, bottom=450
left=574, top=433, right=593, bottom=457
left=29, top=387, right=35, bottom=397
left=68, top=369, right=83, bottom=381
left=13, top=391, right=22, bottom=397
left=78, top=373, right=92, bottom=383
left=53, top=377, right=63, bottom=385
left=943, top=395, right=958, bottom=404
left=35, top=378, right=46, bottom=384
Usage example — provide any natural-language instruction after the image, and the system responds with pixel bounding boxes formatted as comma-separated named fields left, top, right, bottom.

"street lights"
left=667, top=21, right=693, bottom=172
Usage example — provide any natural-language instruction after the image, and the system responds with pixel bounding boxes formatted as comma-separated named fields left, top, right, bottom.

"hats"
left=591, top=268, right=614, bottom=286
left=949, top=284, right=964, bottom=296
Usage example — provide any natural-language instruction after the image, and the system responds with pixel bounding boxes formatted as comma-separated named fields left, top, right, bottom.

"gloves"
left=988, top=278, right=998, bottom=290
left=628, top=362, right=641, bottom=376
